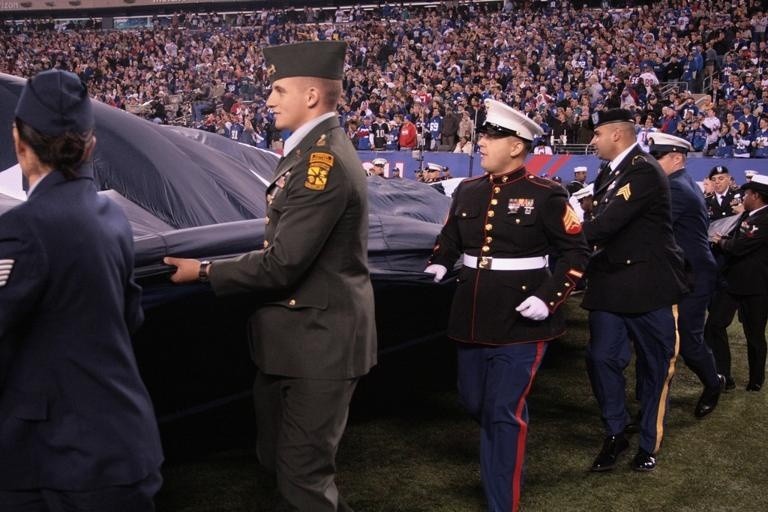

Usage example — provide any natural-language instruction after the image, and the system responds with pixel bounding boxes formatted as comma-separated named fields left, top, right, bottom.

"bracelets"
left=199, top=260, right=210, bottom=282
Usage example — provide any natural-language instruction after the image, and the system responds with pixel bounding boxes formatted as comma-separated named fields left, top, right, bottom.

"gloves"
left=516, top=296, right=550, bottom=321
left=423, top=264, right=447, bottom=283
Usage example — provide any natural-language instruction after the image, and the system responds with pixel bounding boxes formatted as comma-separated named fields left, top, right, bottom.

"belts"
left=462, top=253, right=549, bottom=270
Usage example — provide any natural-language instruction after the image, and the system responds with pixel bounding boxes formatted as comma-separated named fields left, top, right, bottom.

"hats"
left=371, top=158, right=448, bottom=173
left=475, top=98, right=544, bottom=142
left=708, top=165, right=768, bottom=192
left=587, top=110, right=636, bottom=130
left=263, top=41, right=347, bottom=86
left=573, top=167, right=588, bottom=174
left=14, top=70, right=95, bottom=136
left=647, top=132, right=692, bottom=157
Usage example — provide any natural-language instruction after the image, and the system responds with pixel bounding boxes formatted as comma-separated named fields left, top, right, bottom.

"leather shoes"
left=590, top=431, right=630, bottom=472
left=632, top=450, right=658, bottom=472
left=695, top=373, right=765, bottom=416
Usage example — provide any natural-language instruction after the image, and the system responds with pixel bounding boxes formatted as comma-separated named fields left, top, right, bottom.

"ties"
left=597, top=167, right=611, bottom=190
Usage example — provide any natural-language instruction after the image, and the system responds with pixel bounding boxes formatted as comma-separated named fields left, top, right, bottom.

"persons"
left=0, top=67, right=166, bottom=509
left=161, top=38, right=378, bottom=511
left=537, top=185, right=603, bottom=292
left=645, top=131, right=721, bottom=421
left=1, top=0, right=767, bottom=223
left=577, top=106, right=689, bottom=472
left=699, top=165, right=767, bottom=394
left=422, top=97, right=591, bottom=512
